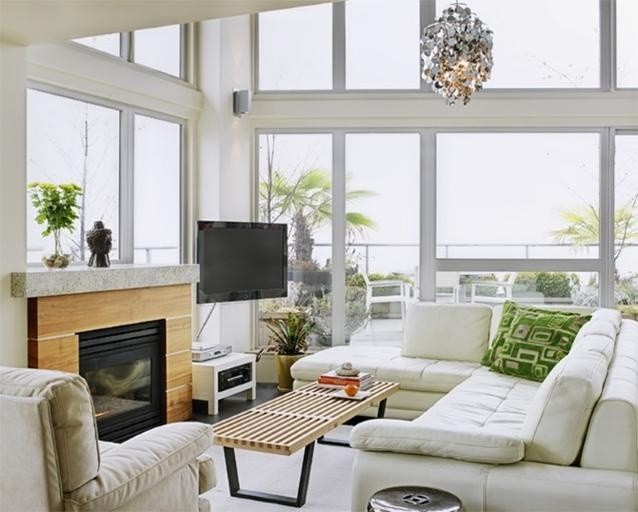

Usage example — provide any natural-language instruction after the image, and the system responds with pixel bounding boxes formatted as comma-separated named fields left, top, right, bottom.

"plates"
left=330, top=388, right=371, bottom=401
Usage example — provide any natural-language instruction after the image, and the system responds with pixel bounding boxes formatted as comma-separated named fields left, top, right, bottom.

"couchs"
left=0, top=362, right=219, bottom=510
left=289, top=300, right=638, bottom=511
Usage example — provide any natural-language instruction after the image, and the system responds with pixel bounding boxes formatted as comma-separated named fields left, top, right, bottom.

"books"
left=318, top=369, right=374, bottom=388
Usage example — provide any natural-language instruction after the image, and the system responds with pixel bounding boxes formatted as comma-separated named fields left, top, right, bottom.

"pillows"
left=482, top=298, right=581, bottom=367
left=488, top=309, right=592, bottom=384
left=401, top=300, right=492, bottom=361
left=349, top=418, right=525, bottom=464
left=0, top=363, right=101, bottom=492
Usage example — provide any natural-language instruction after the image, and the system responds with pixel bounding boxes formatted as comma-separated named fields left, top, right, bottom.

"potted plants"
left=255, top=310, right=318, bottom=393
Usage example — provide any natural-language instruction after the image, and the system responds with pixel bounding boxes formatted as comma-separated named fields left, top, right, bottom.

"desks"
left=191, top=351, right=258, bottom=416
left=209, top=379, right=402, bottom=509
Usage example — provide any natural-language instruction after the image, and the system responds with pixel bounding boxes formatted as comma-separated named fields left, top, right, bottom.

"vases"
left=40, top=232, right=73, bottom=271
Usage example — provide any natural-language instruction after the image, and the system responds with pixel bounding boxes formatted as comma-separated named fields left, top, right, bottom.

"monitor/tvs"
left=195, top=218, right=289, bottom=303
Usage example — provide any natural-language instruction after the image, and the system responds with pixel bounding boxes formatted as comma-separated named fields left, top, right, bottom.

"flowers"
left=27, top=182, right=85, bottom=239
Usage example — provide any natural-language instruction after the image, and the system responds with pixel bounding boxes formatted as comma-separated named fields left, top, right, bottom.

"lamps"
left=417, top=0, right=497, bottom=112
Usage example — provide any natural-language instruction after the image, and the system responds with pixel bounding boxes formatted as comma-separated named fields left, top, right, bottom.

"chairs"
left=360, top=267, right=517, bottom=314
